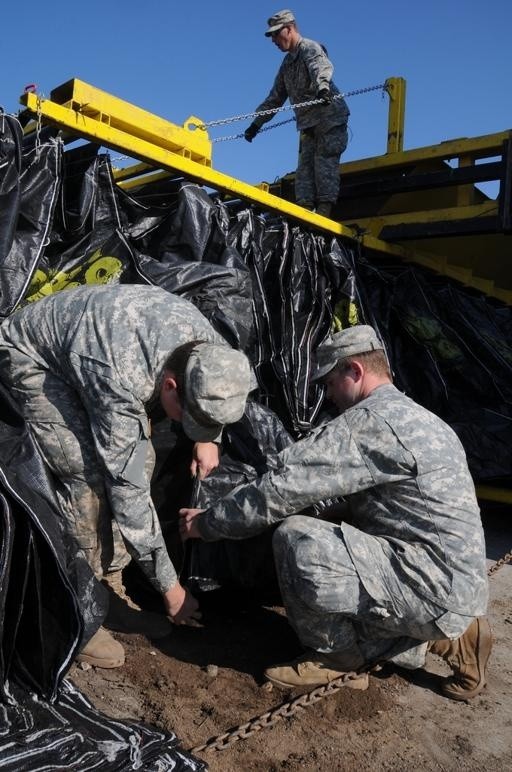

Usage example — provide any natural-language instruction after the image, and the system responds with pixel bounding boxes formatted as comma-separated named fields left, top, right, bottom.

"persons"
left=177, top=324, right=492, bottom=701
left=0, top=285, right=249, bottom=668
left=243, top=9, right=351, bottom=218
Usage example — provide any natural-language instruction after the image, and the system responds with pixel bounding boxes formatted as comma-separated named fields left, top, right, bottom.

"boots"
left=96, top=594, right=171, bottom=636
left=313, top=203, right=332, bottom=218
left=263, top=646, right=368, bottom=693
left=46, top=616, right=125, bottom=669
left=431, top=618, right=491, bottom=699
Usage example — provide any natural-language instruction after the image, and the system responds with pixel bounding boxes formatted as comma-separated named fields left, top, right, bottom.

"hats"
left=307, top=325, right=386, bottom=387
left=263, top=8, right=296, bottom=37
left=177, top=340, right=260, bottom=444
